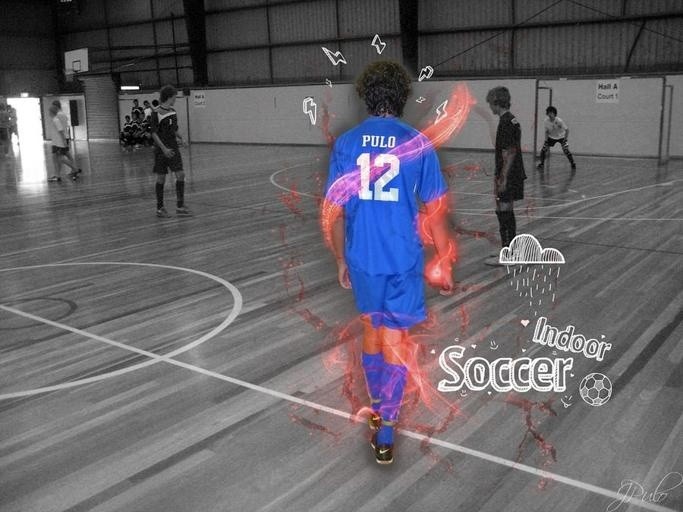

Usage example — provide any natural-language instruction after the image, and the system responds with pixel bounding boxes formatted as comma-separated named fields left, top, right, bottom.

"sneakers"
left=369, top=432, right=394, bottom=466
left=175, top=206, right=193, bottom=216
left=484, top=256, right=514, bottom=266
left=48, top=176, right=60, bottom=182
left=68, top=169, right=81, bottom=176
left=368, top=414, right=381, bottom=430
left=155, top=206, right=172, bottom=218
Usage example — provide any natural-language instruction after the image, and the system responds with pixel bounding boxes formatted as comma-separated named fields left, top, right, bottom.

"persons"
left=149, top=85, right=193, bottom=218
left=46, top=105, right=81, bottom=182
left=119, top=99, right=158, bottom=152
left=322, top=59, right=455, bottom=464
left=51, top=100, right=75, bottom=177
left=0, top=103, right=9, bottom=158
left=535, top=106, right=576, bottom=168
left=6, top=105, right=19, bottom=146
left=482, top=86, right=527, bottom=266
